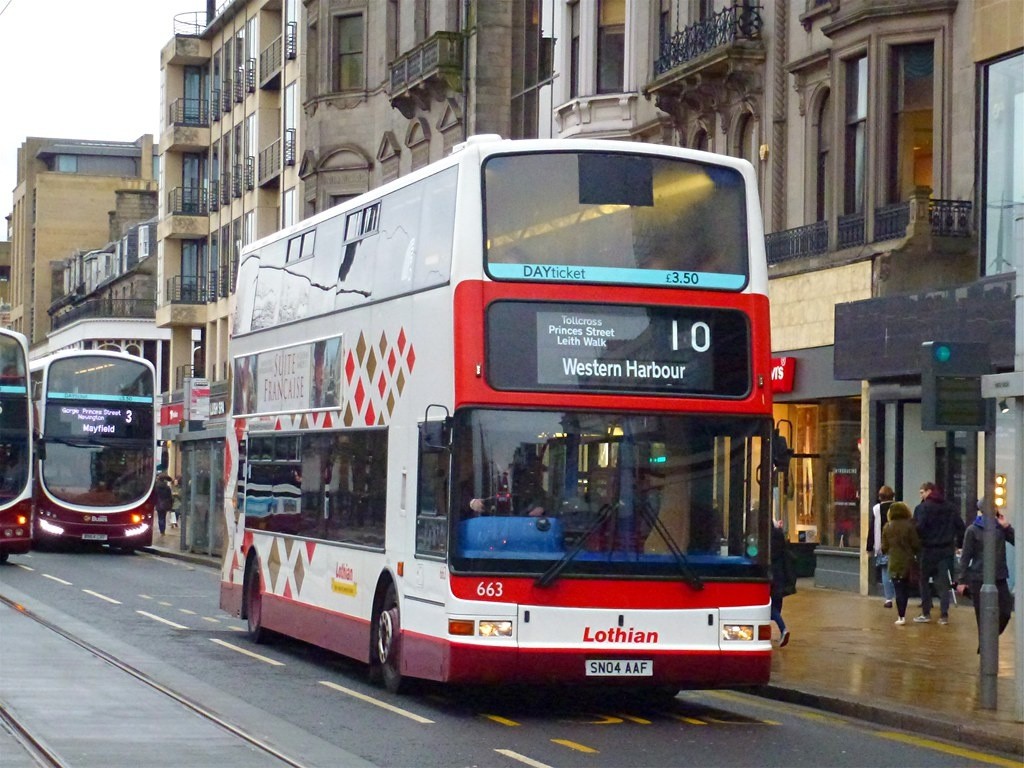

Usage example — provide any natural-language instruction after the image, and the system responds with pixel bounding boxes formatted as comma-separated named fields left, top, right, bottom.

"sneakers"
left=937, top=618, right=949, bottom=625
left=913, top=614, right=932, bottom=623
left=779, top=629, right=790, bottom=647
left=894, top=616, right=906, bottom=625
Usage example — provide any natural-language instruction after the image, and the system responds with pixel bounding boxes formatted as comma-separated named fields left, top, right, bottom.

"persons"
left=881, top=502, right=919, bottom=625
left=911, top=481, right=967, bottom=625
left=771, top=521, right=797, bottom=647
left=153, top=476, right=172, bottom=538
left=169, top=478, right=181, bottom=529
left=777, top=519, right=785, bottom=541
left=460, top=439, right=547, bottom=517
left=866, top=486, right=896, bottom=608
left=954, top=496, right=1014, bottom=655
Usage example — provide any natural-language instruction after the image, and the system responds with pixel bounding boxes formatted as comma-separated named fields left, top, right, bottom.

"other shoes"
left=884, top=601, right=892, bottom=608
left=175, top=524, right=179, bottom=528
left=160, top=531, right=166, bottom=538
left=170, top=525, right=174, bottom=529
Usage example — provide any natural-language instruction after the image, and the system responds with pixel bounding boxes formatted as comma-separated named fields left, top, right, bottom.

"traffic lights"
left=920, top=339, right=994, bottom=432
left=995, top=473, right=1007, bottom=508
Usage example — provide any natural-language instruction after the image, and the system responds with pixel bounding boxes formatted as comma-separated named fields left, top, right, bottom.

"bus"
left=30, top=348, right=165, bottom=553
left=219, top=133, right=795, bottom=695
left=0, top=327, right=34, bottom=563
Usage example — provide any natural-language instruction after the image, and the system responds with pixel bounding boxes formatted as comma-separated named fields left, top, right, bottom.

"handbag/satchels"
left=782, top=548, right=799, bottom=597
left=166, top=510, right=177, bottom=527
left=876, top=547, right=889, bottom=569
